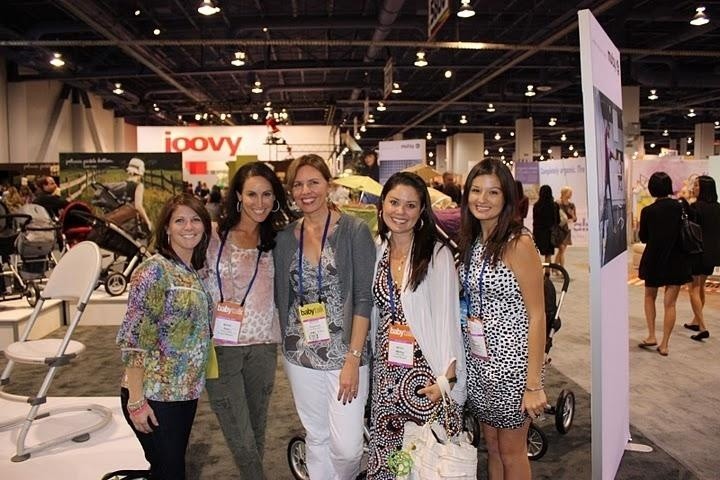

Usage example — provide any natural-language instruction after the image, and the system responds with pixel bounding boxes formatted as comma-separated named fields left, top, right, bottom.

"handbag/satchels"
left=677, top=198, right=703, bottom=255
left=555, top=201, right=568, bottom=227
left=389, top=375, right=477, bottom=480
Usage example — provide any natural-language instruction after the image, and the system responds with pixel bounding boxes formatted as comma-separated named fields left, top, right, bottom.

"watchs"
left=346, top=348, right=362, bottom=359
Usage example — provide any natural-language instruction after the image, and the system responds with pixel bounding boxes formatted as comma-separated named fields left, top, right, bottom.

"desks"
left=1, top=286, right=73, bottom=341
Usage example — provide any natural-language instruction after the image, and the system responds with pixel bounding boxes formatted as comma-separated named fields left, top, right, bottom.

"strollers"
left=91, top=180, right=148, bottom=239
left=0, top=198, right=64, bottom=305
left=71, top=202, right=154, bottom=297
left=55, top=202, right=92, bottom=253
left=463, top=264, right=576, bottom=463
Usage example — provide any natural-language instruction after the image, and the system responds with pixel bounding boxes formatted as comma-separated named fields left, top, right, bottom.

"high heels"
left=551, top=268, right=561, bottom=276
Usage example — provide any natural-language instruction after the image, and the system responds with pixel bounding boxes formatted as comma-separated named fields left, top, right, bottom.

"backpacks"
left=92, top=181, right=126, bottom=209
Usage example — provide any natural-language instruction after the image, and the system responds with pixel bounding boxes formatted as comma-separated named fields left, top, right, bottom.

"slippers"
left=638, top=340, right=657, bottom=347
left=657, top=346, right=668, bottom=357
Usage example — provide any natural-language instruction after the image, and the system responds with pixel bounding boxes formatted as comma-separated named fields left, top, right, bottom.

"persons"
left=684, top=175, right=720, bottom=341
left=115, top=192, right=215, bottom=480
left=531, top=184, right=560, bottom=271
left=188, top=180, right=221, bottom=206
left=196, top=160, right=282, bottom=480
left=456, top=158, right=548, bottom=480
left=434, top=172, right=462, bottom=202
left=554, top=186, right=577, bottom=268
left=123, top=157, right=151, bottom=228
left=33, top=176, right=69, bottom=221
left=371, top=171, right=467, bottom=480
left=361, top=149, right=379, bottom=177
left=637, top=171, right=689, bottom=355
left=273, top=153, right=376, bottom=480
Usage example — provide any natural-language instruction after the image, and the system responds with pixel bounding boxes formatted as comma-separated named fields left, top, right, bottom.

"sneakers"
left=684, top=324, right=699, bottom=331
left=691, top=331, right=709, bottom=340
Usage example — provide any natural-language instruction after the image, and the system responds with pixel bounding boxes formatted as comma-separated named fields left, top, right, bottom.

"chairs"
left=0, top=239, right=112, bottom=457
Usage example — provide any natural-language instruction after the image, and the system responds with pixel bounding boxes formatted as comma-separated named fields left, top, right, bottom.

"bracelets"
left=447, top=376, right=458, bottom=384
left=523, top=386, right=544, bottom=392
left=126, top=397, right=148, bottom=415
